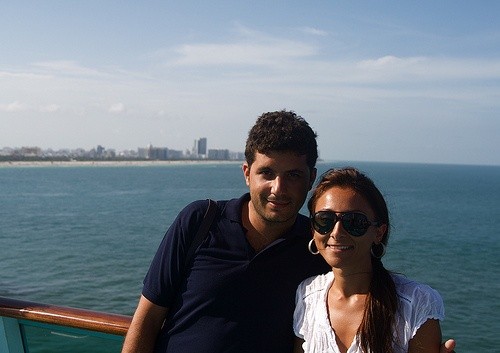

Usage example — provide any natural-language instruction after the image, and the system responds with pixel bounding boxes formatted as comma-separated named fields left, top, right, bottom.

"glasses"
left=310, top=211, right=380, bottom=237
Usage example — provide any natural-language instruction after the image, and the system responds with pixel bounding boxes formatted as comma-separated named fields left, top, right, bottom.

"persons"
left=293, top=169, right=445, bottom=353
left=121, top=110, right=455, bottom=353
